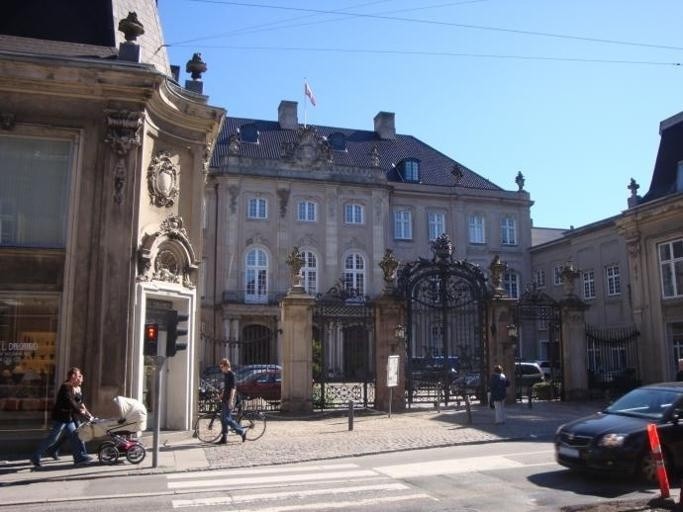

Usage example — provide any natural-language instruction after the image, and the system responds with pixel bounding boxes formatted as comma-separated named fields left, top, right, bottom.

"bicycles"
left=194, top=392, right=265, bottom=444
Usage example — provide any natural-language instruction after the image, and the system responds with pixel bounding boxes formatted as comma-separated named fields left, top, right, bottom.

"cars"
left=200, top=364, right=281, bottom=401
left=553, top=381, right=682, bottom=486
left=405, top=355, right=551, bottom=399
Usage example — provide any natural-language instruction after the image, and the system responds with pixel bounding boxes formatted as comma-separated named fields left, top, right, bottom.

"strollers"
left=74, top=395, right=147, bottom=465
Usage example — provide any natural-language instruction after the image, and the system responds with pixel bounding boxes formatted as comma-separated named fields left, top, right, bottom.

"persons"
left=675, top=358, right=683, bottom=382
left=488, top=365, right=510, bottom=424
left=26, top=366, right=96, bottom=467
left=211, top=358, right=247, bottom=445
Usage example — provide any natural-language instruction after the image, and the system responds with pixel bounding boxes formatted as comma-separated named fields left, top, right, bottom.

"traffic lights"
left=165, top=310, right=187, bottom=357
left=143, top=323, right=158, bottom=356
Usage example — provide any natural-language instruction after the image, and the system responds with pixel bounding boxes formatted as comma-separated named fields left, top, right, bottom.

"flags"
left=304, top=82, right=316, bottom=106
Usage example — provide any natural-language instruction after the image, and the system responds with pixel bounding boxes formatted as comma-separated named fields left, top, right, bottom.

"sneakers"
left=28, top=453, right=43, bottom=468
left=214, top=438, right=227, bottom=445
left=242, top=430, right=247, bottom=442
left=75, top=455, right=92, bottom=467
